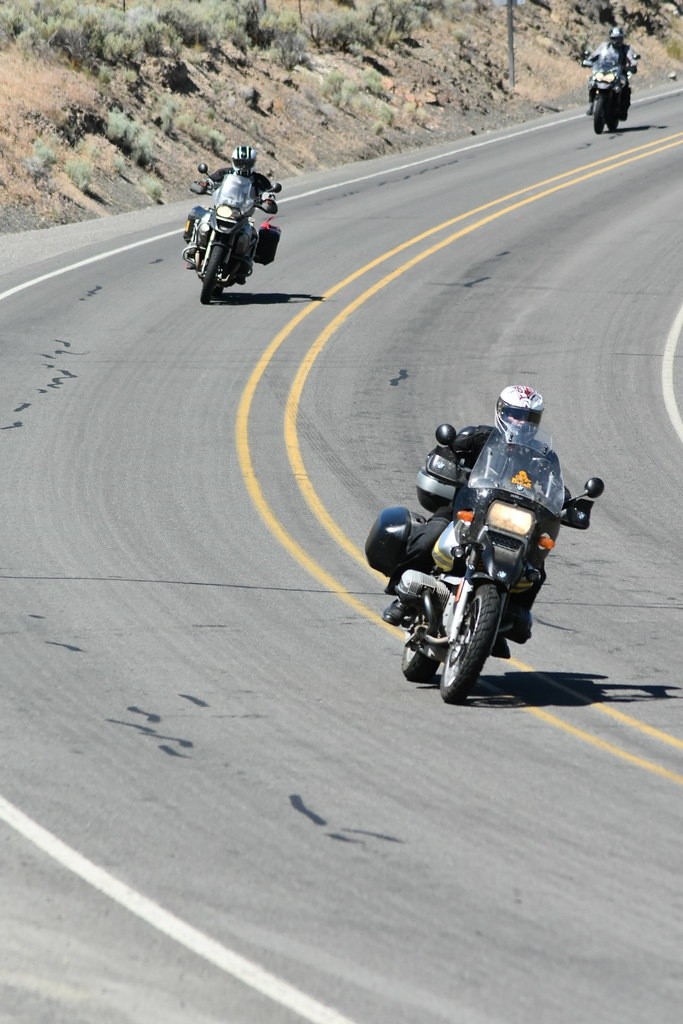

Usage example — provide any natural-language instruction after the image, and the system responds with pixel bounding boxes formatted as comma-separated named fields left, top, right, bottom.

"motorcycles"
left=582, top=48, right=641, bottom=134
left=365, top=418, right=606, bottom=706
left=183, top=163, right=284, bottom=305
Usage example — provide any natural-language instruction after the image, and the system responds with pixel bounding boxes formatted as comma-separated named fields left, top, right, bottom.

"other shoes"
left=185, top=257, right=196, bottom=269
left=237, top=271, right=246, bottom=285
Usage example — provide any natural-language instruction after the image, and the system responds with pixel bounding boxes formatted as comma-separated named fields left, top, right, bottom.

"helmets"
left=494, top=385, right=544, bottom=439
left=231, top=145, right=256, bottom=174
left=609, top=26, right=624, bottom=48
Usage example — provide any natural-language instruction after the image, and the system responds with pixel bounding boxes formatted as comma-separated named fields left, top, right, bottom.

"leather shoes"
left=491, top=636, right=511, bottom=659
left=383, top=598, right=410, bottom=627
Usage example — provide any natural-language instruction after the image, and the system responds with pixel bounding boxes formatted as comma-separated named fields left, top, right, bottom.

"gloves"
left=200, top=181, right=208, bottom=189
left=266, top=199, right=274, bottom=206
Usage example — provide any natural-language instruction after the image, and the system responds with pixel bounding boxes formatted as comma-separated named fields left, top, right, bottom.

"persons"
left=586, top=26, right=637, bottom=121
left=381, top=384, right=589, bottom=644
left=183, top=145, right=276, bottom=285
